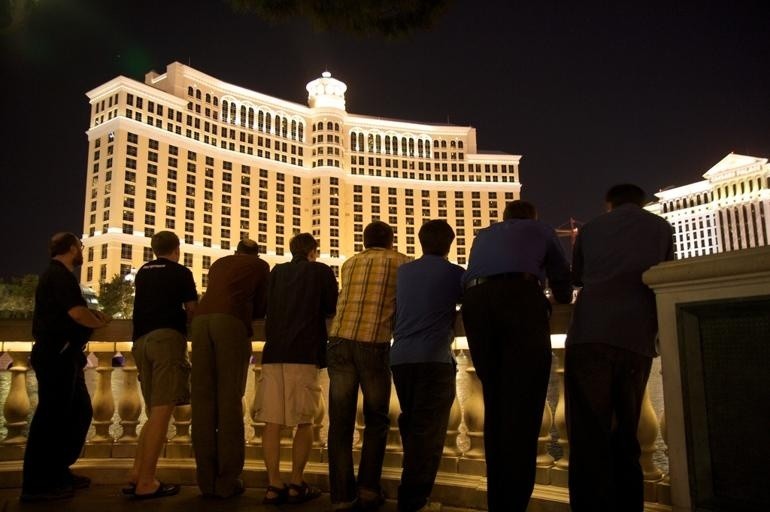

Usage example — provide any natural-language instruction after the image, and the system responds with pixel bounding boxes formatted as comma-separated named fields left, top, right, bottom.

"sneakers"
left=418, top=498, right=443, bottom=512
left=332, top=488, right=385, bottom=511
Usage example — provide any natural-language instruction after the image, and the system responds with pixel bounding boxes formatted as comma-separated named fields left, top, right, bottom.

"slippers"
left=122, top=480, right=179, bottom=498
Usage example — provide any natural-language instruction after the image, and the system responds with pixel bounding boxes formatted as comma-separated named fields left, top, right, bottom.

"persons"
left=459, top=200, right=574, bottom=511
left=565, top=183, right=673, bottom=512
left=252, top=232, right=338, bottom=503
left=189, top=238, right=271, bottom=500
left=123, top=231, right=197, bottom=498
left=327, top=220, right=413, bottom=509
left=19, top=231, right=110, bottom=502
left=391, top=220, right=466, bottom=509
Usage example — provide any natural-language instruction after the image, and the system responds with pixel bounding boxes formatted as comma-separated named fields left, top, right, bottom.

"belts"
left=463, top=273, right=543, bottom=290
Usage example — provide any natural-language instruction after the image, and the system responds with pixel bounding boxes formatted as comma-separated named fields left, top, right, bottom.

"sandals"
left=263, top=482, right=322, bottom=505
